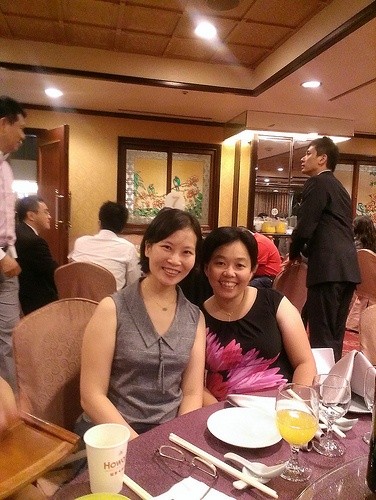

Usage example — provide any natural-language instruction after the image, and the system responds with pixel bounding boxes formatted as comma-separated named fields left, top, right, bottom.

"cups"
left=83, top=423, right=131, bottom=493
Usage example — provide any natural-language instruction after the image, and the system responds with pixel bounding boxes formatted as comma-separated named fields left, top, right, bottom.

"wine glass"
left=275, top=382, right=319, bottom=482
left=310, top=373, right=351, bottom=457
left=362, top=366, right=376, bottom=445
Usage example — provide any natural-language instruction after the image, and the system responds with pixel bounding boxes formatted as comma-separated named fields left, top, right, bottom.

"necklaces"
left=214, top=289, right=245, bottom=319
left=142, top=285, right=171, bottom=312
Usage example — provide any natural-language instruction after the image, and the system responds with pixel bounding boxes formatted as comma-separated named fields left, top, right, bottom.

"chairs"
left=55, top=261, right=118, bottom=301
left=272, top=247, right=376, bottom=365
left=11, top=296, right=101, bottom=493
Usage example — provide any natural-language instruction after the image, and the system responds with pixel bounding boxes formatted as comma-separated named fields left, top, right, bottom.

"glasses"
left=155, top=445, right=218, bottom=479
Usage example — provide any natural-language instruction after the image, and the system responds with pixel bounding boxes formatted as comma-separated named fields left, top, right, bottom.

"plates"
left=206, top=407, right=283, bottom=449
left=349, top=394, right=372, bottom=412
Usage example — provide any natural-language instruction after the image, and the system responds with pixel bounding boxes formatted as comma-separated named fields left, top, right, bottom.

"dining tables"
left=49, top=386, right=376, bottom=500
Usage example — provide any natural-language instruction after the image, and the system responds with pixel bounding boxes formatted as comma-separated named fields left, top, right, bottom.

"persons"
left=68, top=199, right=142, bottom=292
left=239, top=135, right=376, bottom=365
left=198, top=226, right=317, bottom=406
left=0, top=92, right=28, bottom=433
left=14, top=196, right=59, bottom=316
left=72, top=208, right=206, bottom=443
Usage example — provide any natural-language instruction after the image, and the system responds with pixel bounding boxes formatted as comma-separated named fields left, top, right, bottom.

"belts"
left=1, top=247, right=7, bottom=252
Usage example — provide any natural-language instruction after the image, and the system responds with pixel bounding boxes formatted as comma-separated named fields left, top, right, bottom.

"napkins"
left=323, top=350, right=376, bottom=407
left=225, top=394, right=277, bottom=411
left=154, top=474, right=239, bottom=500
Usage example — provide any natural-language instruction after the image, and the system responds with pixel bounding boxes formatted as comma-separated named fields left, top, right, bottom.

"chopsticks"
left=169, top=433, right=278, bottom=499
left=124, top=473, right=154, bottom=500
left=286, top=388, right=345, bottom=437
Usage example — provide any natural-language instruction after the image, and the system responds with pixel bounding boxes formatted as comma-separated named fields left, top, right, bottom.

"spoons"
left=223, top=452, right=289, bottom=478
left=232, top=462, right=272, bottom=490
left=336, top=417, right=358, bottom=427
